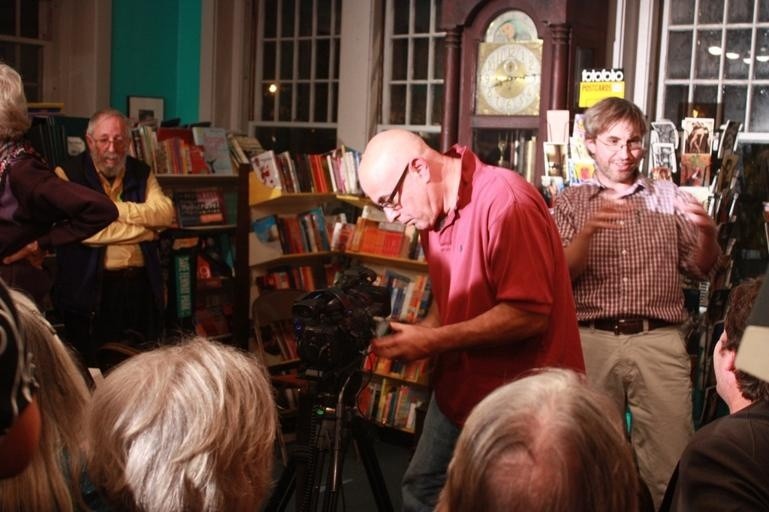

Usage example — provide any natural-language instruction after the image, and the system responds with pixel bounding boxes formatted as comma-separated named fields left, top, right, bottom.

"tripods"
left=266, top=403, right=395, bottom=512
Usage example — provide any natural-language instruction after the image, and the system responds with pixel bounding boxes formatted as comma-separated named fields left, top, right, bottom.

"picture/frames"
left=127, top=96, right=166, bottom=132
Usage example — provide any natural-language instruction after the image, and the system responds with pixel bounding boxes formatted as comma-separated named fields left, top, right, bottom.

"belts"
left=577, top=317, right=672, bottom=334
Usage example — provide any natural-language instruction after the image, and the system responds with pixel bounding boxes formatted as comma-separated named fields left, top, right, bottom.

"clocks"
left=440, top=0, right=609, bottom=200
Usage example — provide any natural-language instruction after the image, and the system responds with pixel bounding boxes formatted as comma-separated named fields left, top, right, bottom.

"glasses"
left=378, top=162, right=410, bottom=213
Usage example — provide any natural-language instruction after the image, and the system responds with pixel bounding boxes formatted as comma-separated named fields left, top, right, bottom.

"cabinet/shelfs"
left=250, top=172, right=433, bottom=458
left=155, top=162, right=250, bottom=350
left=675, top=152, right=753, bottom=421
